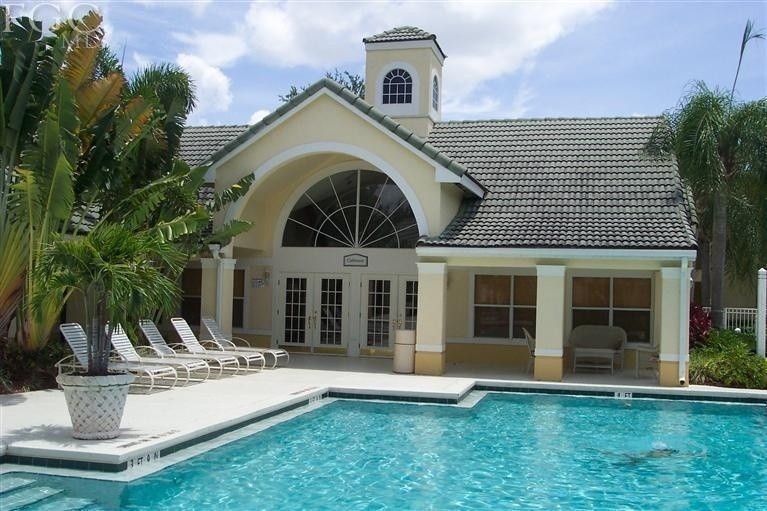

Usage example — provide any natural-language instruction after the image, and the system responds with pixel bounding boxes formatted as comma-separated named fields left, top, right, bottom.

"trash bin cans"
left=392, top=329, right=416, bottom=374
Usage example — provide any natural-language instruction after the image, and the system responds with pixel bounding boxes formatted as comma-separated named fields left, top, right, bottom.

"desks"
left=573, top=348, right=614, bottom=376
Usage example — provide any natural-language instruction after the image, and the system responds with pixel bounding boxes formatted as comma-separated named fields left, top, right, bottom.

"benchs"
left=569, top=326, right=627, bottom=368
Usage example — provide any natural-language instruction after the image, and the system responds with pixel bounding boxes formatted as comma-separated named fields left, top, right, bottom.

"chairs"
left=636, top=342, right=660, bottom=378
left=54, top=317, right=290, bottom=395
left=522, top=328, right=535, bottom=376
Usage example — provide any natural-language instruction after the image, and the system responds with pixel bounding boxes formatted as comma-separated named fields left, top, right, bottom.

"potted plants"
left=17, top=221, right=189, bottom=440
